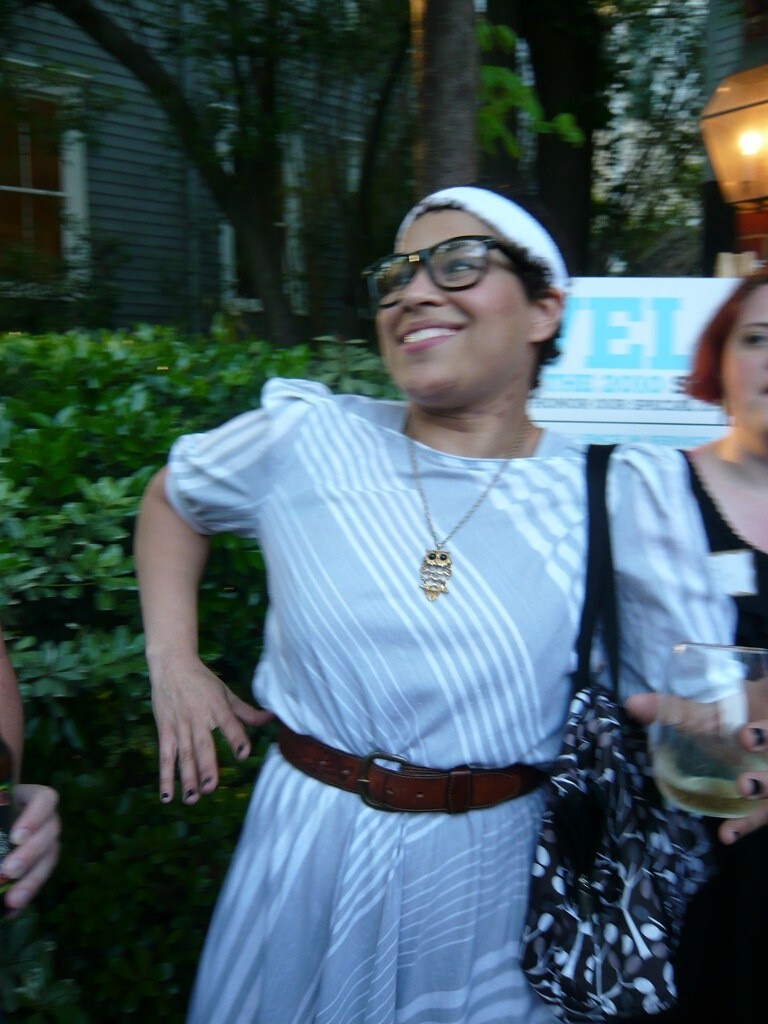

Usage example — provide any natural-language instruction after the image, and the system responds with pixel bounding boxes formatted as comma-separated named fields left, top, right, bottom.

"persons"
left=0, top=626, right=63, bottom=922
left=132, top=182, right=767, bottom=1023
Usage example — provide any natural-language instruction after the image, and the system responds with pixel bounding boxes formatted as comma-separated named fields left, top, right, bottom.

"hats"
left=397, top=186, right=569, bottom=293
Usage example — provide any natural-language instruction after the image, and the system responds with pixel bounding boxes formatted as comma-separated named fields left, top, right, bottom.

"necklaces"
left=407, top=415, right=534, bottom=603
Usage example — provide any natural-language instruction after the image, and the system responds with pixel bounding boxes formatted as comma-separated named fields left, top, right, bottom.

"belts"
left=274, top=718, right=542, bottom=816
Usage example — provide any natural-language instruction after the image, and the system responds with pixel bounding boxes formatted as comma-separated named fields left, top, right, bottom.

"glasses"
left=359, top=235, right=533, bottom=310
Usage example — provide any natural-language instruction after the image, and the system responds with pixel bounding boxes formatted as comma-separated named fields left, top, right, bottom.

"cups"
left=654, top=643, right=768, bottom=818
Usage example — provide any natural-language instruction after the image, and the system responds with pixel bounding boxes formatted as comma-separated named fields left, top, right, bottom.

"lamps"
left=697, top=63, right=768, bottom=215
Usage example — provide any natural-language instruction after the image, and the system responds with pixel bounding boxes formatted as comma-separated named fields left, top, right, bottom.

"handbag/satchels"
left=521, top=444, right=712, bottom=1024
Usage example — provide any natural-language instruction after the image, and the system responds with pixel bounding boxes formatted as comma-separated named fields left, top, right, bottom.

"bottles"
left=0, top=737, right=53, bottom=1014
